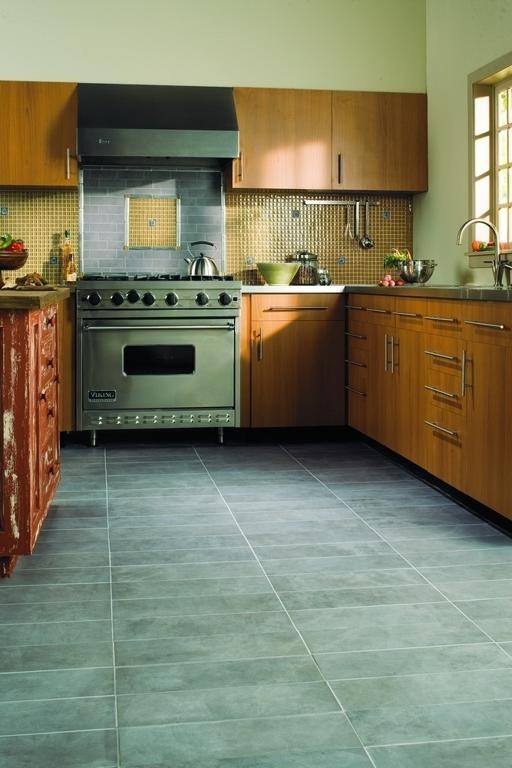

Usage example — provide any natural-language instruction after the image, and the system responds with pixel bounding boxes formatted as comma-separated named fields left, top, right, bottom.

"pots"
left=396, top=258, right=439, bottom=285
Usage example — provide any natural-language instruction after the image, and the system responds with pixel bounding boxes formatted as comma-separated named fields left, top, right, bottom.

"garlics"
left=377, top=274, right=396, bottom=287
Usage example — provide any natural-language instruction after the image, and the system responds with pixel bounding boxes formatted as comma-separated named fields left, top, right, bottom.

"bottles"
left=61, top=228, right=77, bottom=282
left=296, top=252, right=319, bottom=285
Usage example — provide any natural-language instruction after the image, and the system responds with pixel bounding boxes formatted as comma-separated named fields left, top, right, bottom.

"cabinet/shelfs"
left=0, top=80, right=78, bottom=187
left=343, top=292, right=365, bottom=436
left=0, top=299, right=60, bottom=577
left=460, top=299, right=511, bottom=520
left=249, top=293, right=346, bottom=427
left=231, top=87, right=330, bottom=189
left=426, top=297, right=460, bottom=491
left=365, top=294, right=426, bottom=478
left=329, top=90, right=427, bottom=191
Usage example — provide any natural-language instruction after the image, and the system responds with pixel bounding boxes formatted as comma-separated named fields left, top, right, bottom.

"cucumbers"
left=0, top=233, right=12, bottom=250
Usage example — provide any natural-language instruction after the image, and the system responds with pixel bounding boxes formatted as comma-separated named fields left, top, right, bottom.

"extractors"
left=75, top=83, right=242, bottom=161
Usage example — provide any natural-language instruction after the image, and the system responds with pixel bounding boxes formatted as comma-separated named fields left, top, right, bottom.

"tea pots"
left=184, top=240, right=220, bottom=276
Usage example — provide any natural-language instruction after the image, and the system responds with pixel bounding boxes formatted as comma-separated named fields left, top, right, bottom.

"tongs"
left=353, top=199, right=361, bottom=241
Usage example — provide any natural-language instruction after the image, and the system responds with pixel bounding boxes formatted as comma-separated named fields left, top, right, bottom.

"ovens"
left=76, top=281, right=241, bottom=433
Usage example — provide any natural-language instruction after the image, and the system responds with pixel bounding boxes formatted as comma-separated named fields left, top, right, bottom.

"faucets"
left=457, top=218, right=500, bottom=269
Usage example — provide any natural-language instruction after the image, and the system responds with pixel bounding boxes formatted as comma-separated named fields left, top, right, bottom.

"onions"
left=396, top=279, right=405, bottom=285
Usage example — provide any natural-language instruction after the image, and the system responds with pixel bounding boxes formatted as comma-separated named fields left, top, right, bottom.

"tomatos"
left=7, top=238, right=24, bottom=252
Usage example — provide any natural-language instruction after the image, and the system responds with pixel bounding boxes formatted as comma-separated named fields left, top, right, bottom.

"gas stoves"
left=76, top=273, right=236, bottom=281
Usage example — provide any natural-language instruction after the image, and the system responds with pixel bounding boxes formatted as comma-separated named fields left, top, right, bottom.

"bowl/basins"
left=256, top=261, right=302, bottom=286
